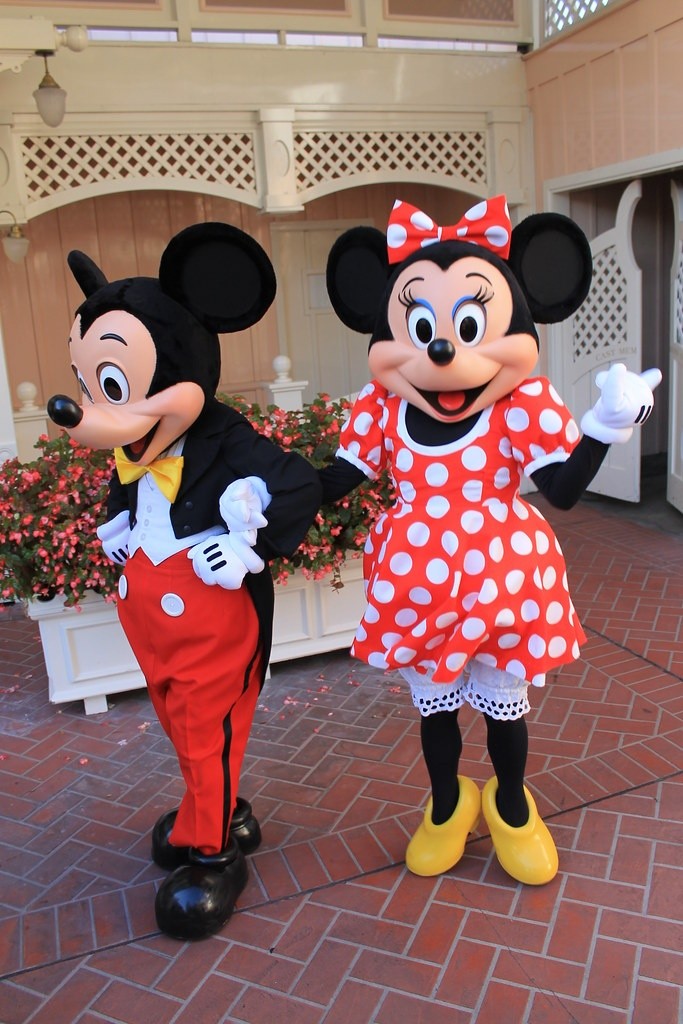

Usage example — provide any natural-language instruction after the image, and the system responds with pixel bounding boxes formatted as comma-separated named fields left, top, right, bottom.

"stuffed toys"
left=47, top=220, right=319, bottom=940
left=216, top=196, right=662, bottom=886
